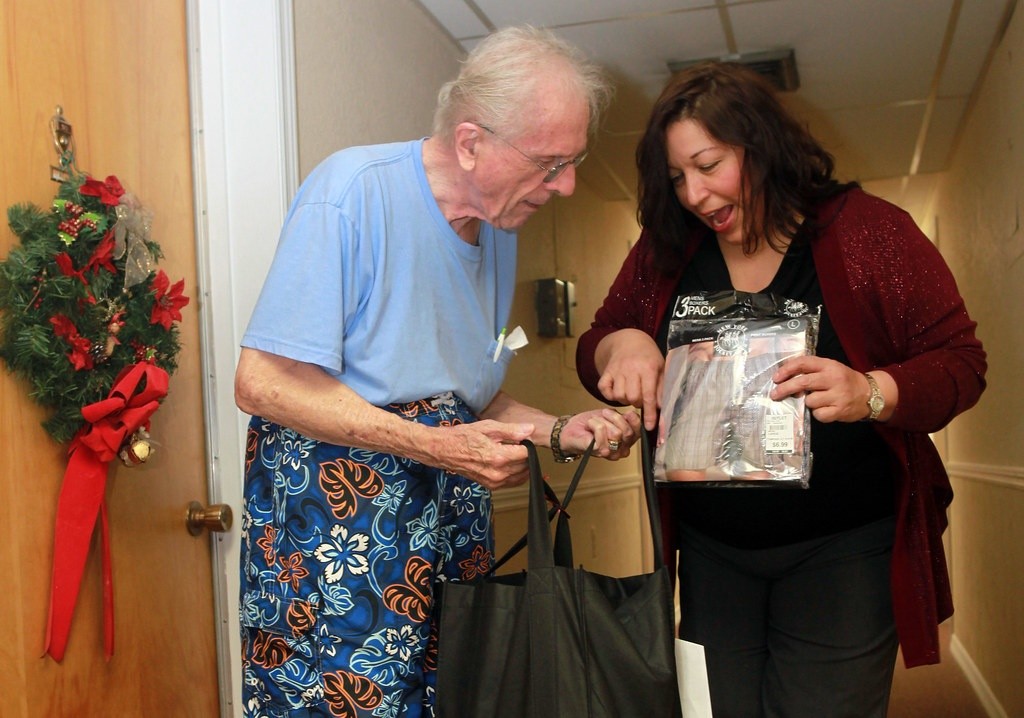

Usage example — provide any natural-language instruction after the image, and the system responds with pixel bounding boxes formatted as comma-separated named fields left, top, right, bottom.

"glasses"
left=476, top=123, right=589, bottom=182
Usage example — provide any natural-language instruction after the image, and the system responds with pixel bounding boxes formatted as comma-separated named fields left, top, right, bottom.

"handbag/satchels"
left=434, top=413, right=684, bottom=718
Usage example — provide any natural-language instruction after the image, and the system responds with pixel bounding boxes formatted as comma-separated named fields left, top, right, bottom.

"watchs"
left=859, top=374, right=886, bottom=422
left=551, top=414, right=581, bottom=464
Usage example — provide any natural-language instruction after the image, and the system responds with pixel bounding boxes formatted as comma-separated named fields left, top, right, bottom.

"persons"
left=573, top=61, right=989, bottom=718
left=238, top=21, right=643, bottom=718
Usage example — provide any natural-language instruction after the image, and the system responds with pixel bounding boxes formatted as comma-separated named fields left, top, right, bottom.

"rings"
left=608, top=439, right=622, bottom=453
